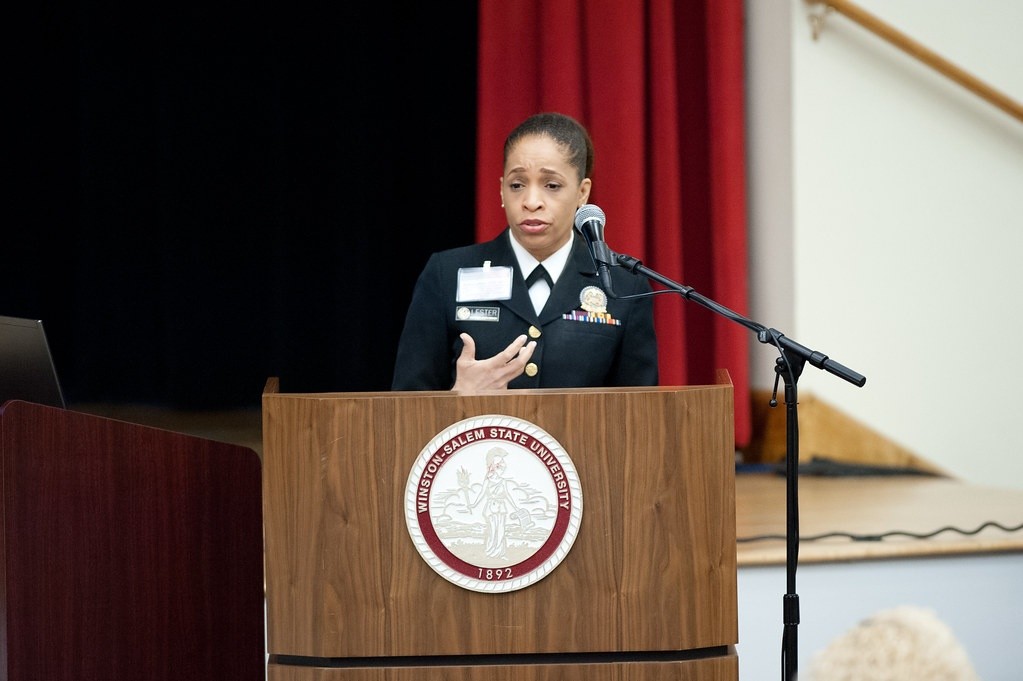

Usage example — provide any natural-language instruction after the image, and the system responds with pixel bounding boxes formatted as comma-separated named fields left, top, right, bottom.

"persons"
left=392, top=116, right=659, bottom=391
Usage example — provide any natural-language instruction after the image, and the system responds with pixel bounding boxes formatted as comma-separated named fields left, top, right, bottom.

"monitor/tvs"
left=0, top=316, right=68, bottom=410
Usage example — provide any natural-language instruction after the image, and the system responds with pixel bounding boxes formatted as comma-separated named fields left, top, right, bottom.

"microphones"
left=575, top=204, right=618, bottom=299
